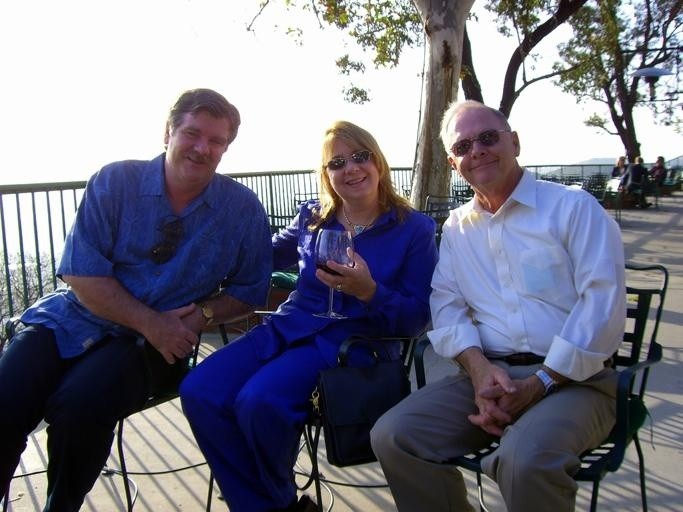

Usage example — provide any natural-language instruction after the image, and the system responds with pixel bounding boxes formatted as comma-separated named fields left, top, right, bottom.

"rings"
left=336, top=283, right=343, bottom=292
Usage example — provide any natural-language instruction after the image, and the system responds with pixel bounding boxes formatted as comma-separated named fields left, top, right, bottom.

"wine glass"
left=312, top=229, right=356, bottom=320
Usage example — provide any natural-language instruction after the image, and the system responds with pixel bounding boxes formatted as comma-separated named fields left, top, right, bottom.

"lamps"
left=628, top=67, right=679, bottom=101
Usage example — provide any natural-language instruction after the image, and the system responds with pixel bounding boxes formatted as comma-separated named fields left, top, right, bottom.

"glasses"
left=148, top=216, right=184, bottom=264
left=450, top=128, right=510, bottom=158
left=325, top=149, right=373, bottom=171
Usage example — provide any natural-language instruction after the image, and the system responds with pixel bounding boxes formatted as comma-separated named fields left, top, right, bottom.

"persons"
left=610, top=155, right=667, bottom=208
left=180, top=121, right=440, bottom=511
left=370, top=98, right=627, bottom=511
left=0, top=90, right=274, bottom=512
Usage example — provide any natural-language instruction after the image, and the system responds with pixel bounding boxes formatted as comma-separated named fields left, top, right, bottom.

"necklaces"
left=341, top=208, right=372, bottom=236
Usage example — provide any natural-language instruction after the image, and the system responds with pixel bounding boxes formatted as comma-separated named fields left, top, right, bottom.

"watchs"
left=535, top=369, right=560, bottom=396
left=197, top=302, right=215, bottom=327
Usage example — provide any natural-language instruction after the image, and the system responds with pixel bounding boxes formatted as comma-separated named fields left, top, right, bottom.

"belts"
left=485, top=350, right=546, bottom=366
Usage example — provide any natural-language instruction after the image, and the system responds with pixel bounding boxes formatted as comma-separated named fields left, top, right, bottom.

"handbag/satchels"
left=310, top=331, right=413, bottom=468
